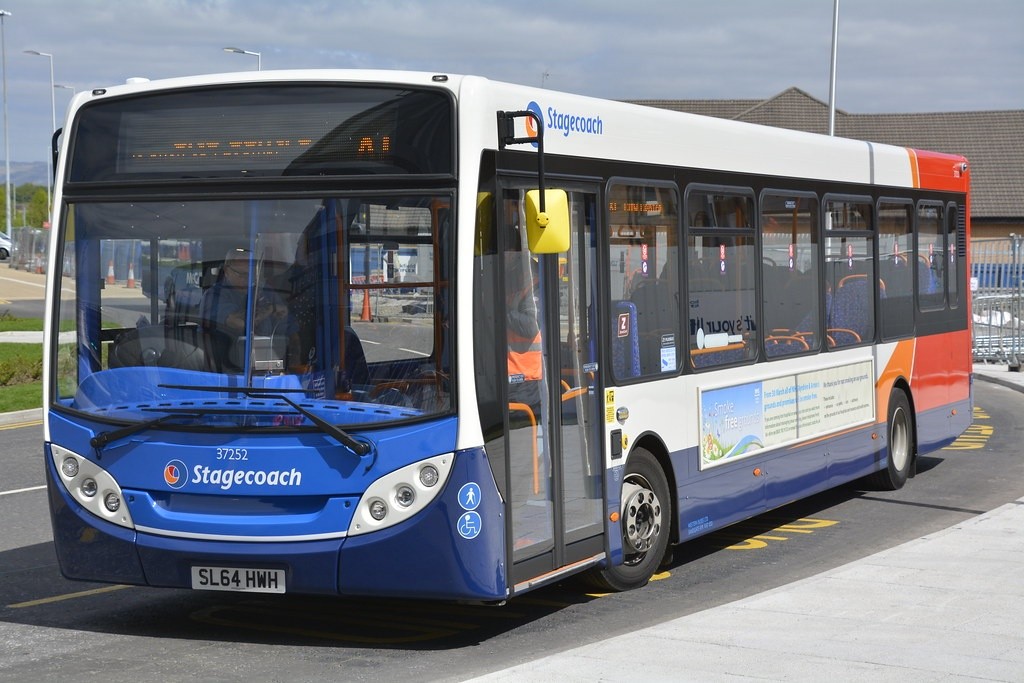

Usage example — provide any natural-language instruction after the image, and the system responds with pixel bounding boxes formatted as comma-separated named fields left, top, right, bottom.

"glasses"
left=226, top=265, right=250, bottom=279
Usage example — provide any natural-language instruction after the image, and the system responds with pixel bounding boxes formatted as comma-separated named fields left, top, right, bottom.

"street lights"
left=222, top=46, right=261, bottom=72
left=0, top=9, right=12, bottom=236
left=54, top=84, right=75, bottom=94
left=22, top=49, right=56, bottom=223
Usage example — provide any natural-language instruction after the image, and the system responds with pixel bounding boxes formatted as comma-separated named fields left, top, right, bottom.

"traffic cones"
left=106, top=260, right=115, bottom=285
left=35, top=256, right=41, bottom=274
left=127, top=262, right=135, bottom=288
left=360, top=289, right=373, bottom=322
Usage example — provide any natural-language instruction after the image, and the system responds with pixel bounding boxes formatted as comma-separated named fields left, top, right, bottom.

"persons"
left=199, top=249, right=298, bottom=370
left=288, top=294, right=373, bottom=399
left=503, top=228, right=544, bottom=429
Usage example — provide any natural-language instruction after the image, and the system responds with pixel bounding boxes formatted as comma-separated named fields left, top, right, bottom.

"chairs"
left=589, top=252, right=932, bottom=378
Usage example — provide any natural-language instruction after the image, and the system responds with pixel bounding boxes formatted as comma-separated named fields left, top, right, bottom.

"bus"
left=40, top=67, right=971, bottom=606
left=139, top=238, right=204, bottom=311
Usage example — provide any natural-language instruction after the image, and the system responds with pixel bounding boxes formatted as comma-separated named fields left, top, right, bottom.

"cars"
left=0, top=231, right=21, bottom=261
